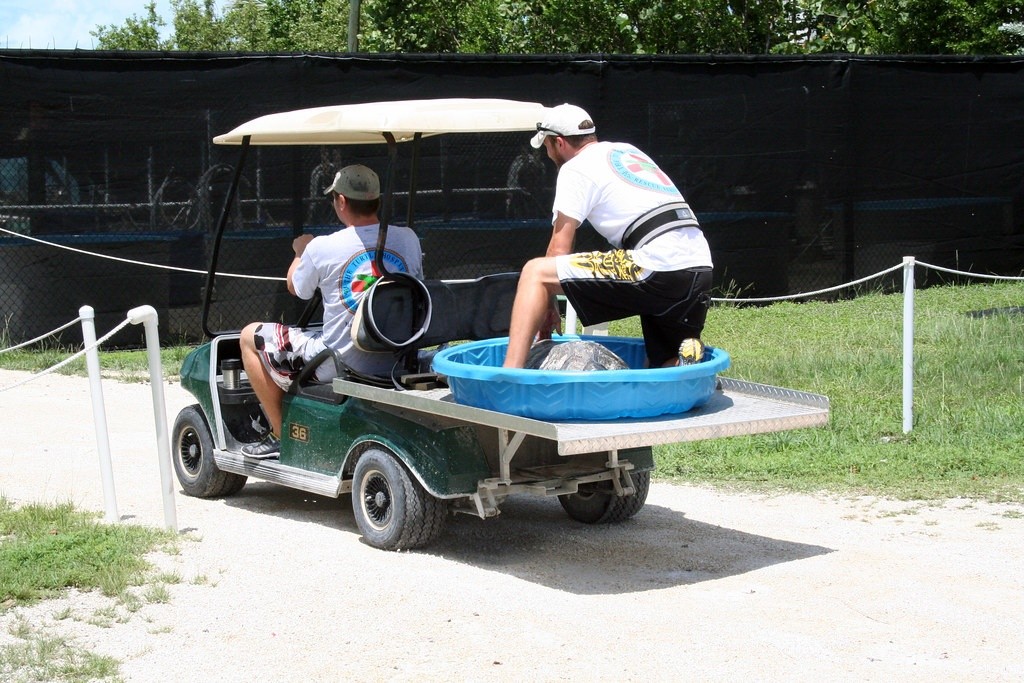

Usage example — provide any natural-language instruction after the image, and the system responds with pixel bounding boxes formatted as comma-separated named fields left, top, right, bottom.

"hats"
left=530, top=102, right=595, bottom=148
left=323, top=164, right=380, bottom=200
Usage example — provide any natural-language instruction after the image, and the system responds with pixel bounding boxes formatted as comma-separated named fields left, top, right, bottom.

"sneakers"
left=240, top=430, right=280, bottom=459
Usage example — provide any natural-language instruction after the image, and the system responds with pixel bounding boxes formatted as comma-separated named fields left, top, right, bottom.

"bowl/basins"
left=433, top=334, right=730, bottom=421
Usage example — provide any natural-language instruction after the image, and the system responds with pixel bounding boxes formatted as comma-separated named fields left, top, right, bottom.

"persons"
left=306, top=147, right=345, bottom=225
left=503, top=104, right=713, bottom=369
left=506, top=141, right=547, bottom=216
left=240, top=165, right=423, bottom=457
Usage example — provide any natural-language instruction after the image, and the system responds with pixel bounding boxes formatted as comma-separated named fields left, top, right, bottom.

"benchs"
left=296, top=272, right=552, bottom=404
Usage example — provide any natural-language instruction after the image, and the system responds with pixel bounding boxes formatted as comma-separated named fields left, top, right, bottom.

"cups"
left=220, top=359, right=242, bottom=389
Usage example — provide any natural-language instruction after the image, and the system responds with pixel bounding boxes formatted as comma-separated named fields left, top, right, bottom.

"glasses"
left=536, top=122, right=564, bottom=138
